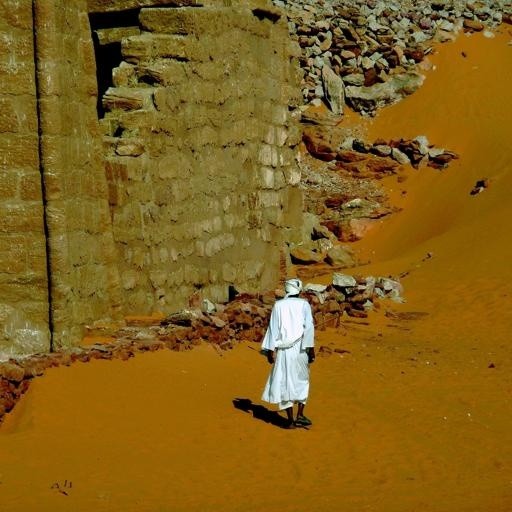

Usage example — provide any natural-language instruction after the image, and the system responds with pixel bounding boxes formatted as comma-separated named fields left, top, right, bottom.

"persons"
left=259, top=279, right=317, bottom=430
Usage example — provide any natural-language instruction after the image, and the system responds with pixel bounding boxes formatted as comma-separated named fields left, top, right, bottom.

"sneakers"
left=295, top=414, right=312, bottom=425
